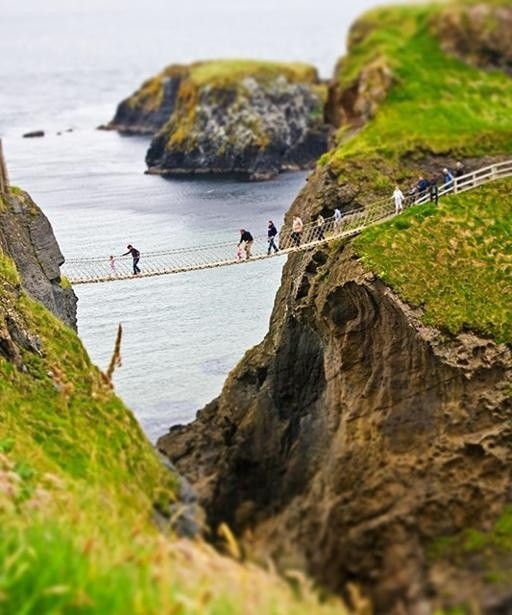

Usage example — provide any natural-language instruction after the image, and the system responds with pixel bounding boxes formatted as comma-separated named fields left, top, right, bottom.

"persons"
left=438, top=167, right=454, bottom=197
left=289, top=215, right=303, bottom=247
left=417, top=176, right=429, bottom=203
left=238, top=229, right=253, bottom=260
left=315, top=214, right=325, bottom=241
left=265, top=220, right=279, bottom=255
left=392, top=187, right=404, bottom=215
left=331, top=207, right=343, bottom=237
left=454, top=161, right=466, bottom=190
left=108, top=256, right=117, bottom=275
left=428, top=174, right=438, bottom=205
left=236, top=243, right=244, bottom=260
left=409, top=184, right=420, bottom=208
left=120, top=245, right=141, bottom=275
